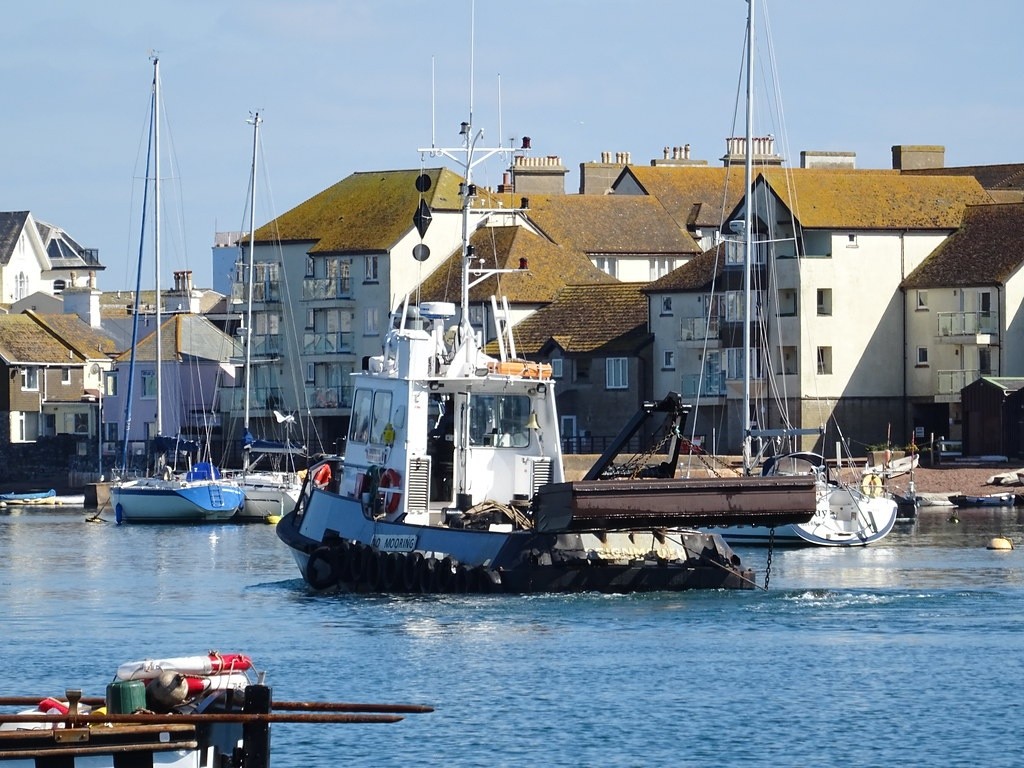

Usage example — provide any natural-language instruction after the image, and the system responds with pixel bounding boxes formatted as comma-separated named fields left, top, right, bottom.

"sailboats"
left=683, top=2, right=921, bottom=546
left=110, top=50, right=245, bottom=522
left=206, top=108, right=325, bottom=519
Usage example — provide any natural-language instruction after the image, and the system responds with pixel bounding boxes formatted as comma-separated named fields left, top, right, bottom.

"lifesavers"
left=311, top=463, right=331, bottom=492
left=380, top=467, right=400, bottom=513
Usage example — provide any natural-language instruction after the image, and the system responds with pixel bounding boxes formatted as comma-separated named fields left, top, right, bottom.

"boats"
left=948, top=495, right=1016, bottom=507
left=277, top=1, right=757, bottom=594
left=0, top=489, right=57, bottom=505
left=920, top=491, right=962, bottom=506
